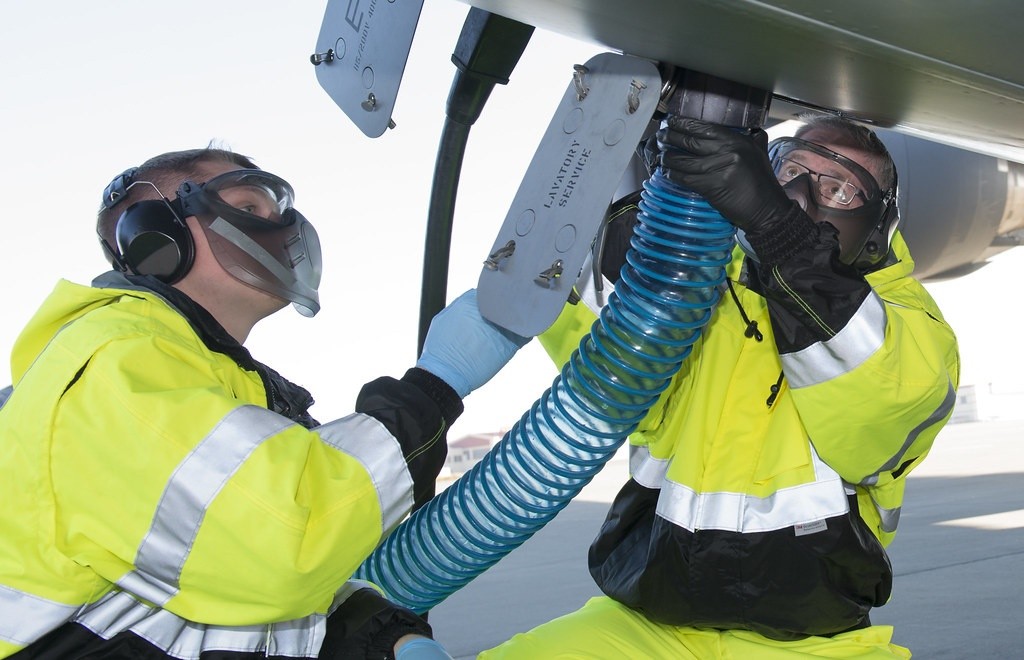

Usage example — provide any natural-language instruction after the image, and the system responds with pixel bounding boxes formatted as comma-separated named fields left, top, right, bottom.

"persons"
left=0, top=143, right=533, bottom=660
left=474, top=115, right=965, bottom=659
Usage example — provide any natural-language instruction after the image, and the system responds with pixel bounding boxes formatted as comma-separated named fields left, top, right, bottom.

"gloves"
left=415, top=292, right=531, bottom=401
left=396, top=638, right=452, bottom=660
left=653, top=111, right=791, bottom=234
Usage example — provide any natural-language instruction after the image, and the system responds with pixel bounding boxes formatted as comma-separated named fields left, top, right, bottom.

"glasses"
left=197, top=169, right=296, bottom=232
left=773, top=157, right=871, bottom=207
